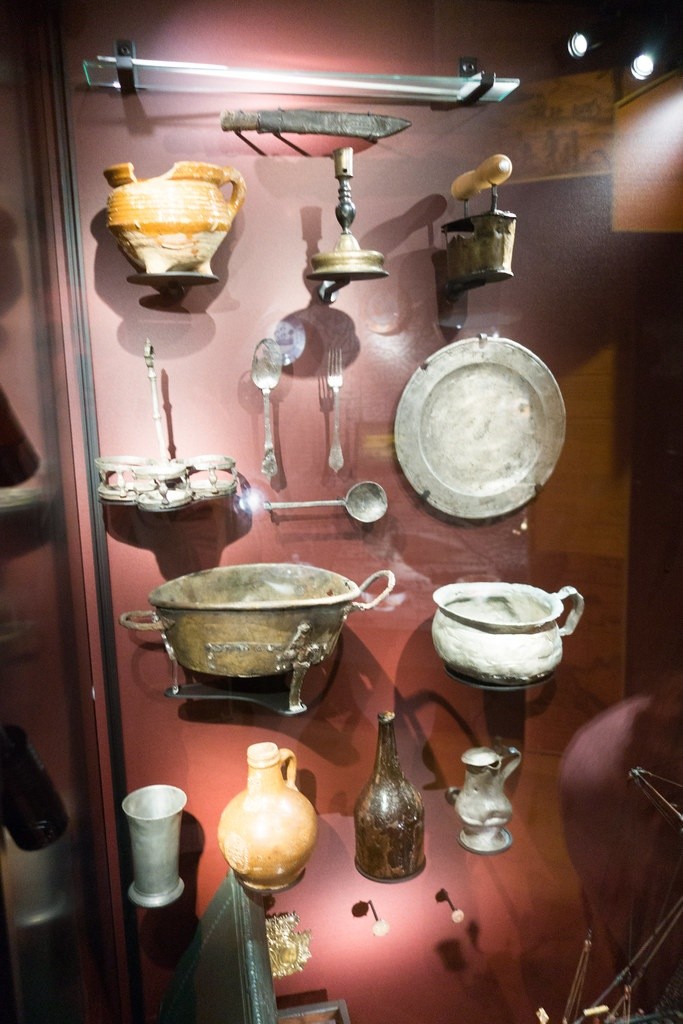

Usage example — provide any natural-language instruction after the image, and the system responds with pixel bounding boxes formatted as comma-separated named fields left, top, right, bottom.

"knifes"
left=220, top=109, right=410, bottom=138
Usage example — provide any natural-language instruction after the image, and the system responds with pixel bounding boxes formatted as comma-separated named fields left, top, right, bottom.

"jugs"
left=431, top=582, right=585, bottom=686
left=456, top=746, right=522, bottom=854
left=218, top=742, right=318, bottom=893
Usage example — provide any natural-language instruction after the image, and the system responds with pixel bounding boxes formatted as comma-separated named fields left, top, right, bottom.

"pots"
left=119, top=563, right=396, bottom=711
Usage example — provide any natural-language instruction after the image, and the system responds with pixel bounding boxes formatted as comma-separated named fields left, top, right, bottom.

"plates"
left=394, top=337, right=566, bottom=519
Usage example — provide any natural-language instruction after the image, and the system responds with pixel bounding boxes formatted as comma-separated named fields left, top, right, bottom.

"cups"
left=122, top=784, right=184, bottom=908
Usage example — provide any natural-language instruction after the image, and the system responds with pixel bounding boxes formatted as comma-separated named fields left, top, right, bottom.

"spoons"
left=251, top=338, right=283, bottom=476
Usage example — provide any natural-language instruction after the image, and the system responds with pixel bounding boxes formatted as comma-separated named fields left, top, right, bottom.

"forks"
left=327, top=347, right=345, bottom=474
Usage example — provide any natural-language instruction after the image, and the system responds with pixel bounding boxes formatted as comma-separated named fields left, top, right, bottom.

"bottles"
left=355, top=711, right=426, bottom=884
